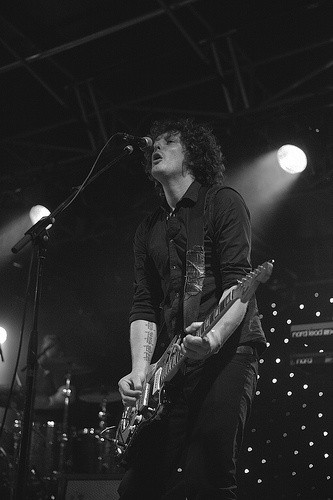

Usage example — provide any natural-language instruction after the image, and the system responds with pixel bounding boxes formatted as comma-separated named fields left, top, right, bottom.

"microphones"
left=124, top=132, right=153, bottom=147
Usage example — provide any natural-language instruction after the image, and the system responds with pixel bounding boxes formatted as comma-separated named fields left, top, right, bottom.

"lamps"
left=276, top=128, right=327, bottom=175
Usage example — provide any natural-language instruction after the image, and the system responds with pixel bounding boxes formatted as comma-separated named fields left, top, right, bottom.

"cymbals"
left=76, top=387, right=121, bottom=403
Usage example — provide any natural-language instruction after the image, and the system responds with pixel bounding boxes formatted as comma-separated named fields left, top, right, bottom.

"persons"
left=118, top=119, right=267, bottom=500
left=17, top=335, right=75, bottom=478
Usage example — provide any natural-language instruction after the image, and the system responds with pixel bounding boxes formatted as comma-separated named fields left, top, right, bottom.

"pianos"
left=291, top=356, right=333, bottom=380
left=289, top=322, right=333, bottom=355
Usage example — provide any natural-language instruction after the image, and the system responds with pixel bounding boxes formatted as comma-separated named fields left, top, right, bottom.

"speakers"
left=58, top=473, right=122, bottom=500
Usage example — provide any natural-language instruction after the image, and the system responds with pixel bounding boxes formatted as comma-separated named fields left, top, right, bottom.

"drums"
left=79, top=425, right=123, bottom=473
left=14, top=420, right=81, bottom=487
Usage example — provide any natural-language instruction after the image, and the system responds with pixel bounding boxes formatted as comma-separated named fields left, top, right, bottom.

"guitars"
left=111, top=258, right=273, bottom=470
